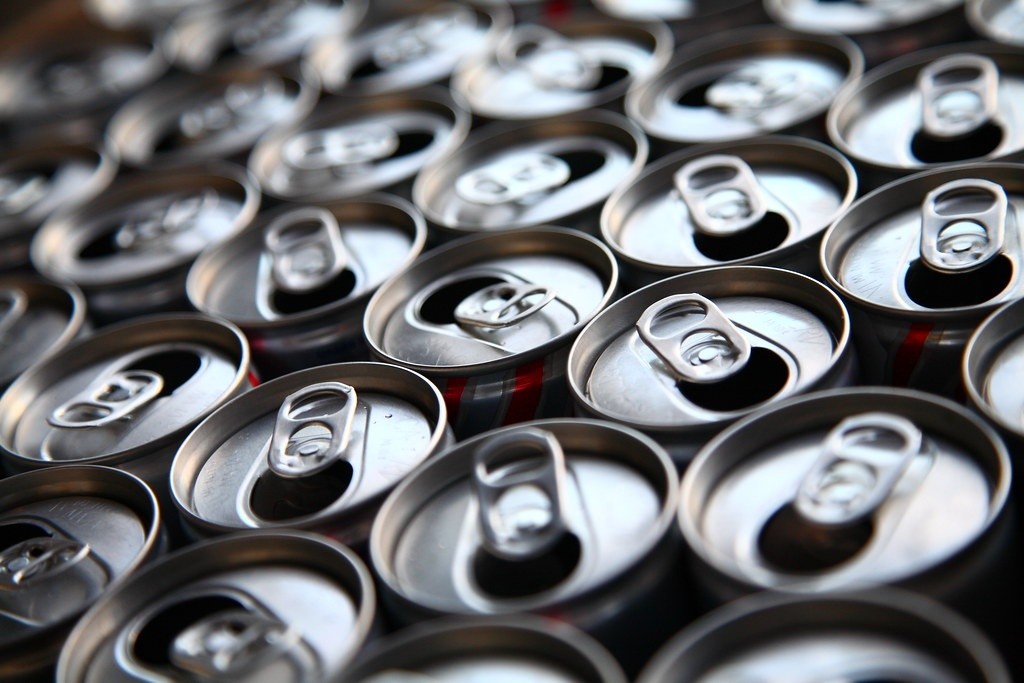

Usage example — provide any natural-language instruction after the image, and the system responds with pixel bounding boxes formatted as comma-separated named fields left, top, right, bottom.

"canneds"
left=0, top=0, right=1024, bottom=683
